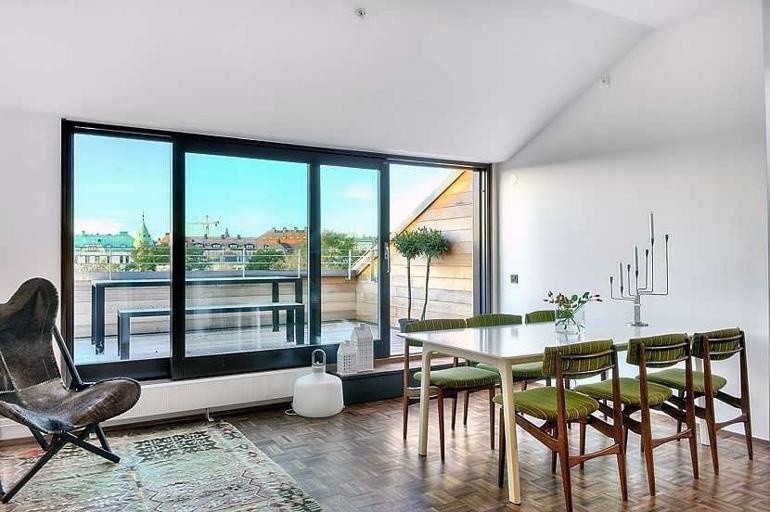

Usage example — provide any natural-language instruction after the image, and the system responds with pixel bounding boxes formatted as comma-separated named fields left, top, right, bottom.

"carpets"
left=0, top=418, right=325, bottom=510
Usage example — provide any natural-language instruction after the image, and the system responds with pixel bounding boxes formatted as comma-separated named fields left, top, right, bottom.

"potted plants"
left=393, top=230, right=420, bottom=334
left=543, top=289, right=605, bottom=331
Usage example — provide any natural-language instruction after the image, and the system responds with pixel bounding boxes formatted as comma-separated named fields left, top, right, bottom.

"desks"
left=397, top=320, right=715, bottom=504
left=90, top=276, right=306, bottom=358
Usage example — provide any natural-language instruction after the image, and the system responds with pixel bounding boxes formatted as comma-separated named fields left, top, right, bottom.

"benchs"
left=116, top=301, right=306, bottom=362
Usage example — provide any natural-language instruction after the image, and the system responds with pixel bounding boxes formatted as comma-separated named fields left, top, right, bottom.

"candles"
left=619, top=261, right=624, bottom=287
left=650, top=212, right=656, bottom=239
left=634, top=245, right=638, bottom=270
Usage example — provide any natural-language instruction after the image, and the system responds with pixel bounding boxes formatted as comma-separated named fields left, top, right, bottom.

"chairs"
left=635, top=327, right=753, bottom=475
left=494, top=338, right=629, bottom=511
left=398, top=309, right=607, bottom=460
left=0, top=277, right=141, bottom=504
left=572, top=333, right=699, bottom=497
